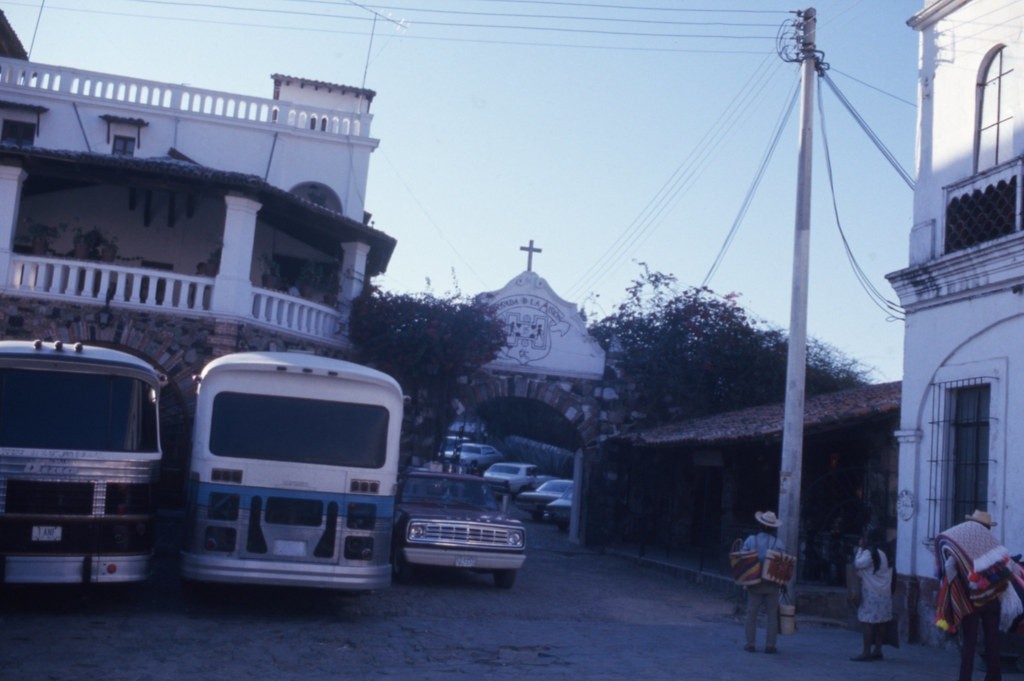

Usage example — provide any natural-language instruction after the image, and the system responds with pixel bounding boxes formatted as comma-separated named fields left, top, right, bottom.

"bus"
left=178, top=350, right=410, bottom=595
left=1, top=337, right=168, bottom=588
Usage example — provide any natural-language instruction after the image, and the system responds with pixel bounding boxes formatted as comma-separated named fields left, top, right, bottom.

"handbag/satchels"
left=763, top=548, right=797, bottom=584
left=871, top=613, right=900, bottom=647
left=728, top=538, right=762, bottom=586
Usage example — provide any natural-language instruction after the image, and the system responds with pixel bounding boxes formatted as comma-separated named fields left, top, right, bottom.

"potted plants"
left=260, top=256, right=283, bottom=292
left=15, top=216, right=68, bottom=255
left=69, top=217, right=119, bottom=262
left=320, top=280, right=343, bottom=306
left=205, top=247, right=222, bottom=277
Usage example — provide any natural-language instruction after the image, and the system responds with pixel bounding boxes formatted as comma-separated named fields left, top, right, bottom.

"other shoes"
left=765, top=646, right=777, bottom=652
left=872, top=652, right=883, bottom=660
left=850, top=654, right=871, bottom=661
left=745, top=644, right=756, bottom=652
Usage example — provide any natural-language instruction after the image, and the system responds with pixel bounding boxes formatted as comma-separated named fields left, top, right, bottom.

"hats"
left=965, top=510, right=997, bottom=527
left=756, top=511, right=783, bottom=527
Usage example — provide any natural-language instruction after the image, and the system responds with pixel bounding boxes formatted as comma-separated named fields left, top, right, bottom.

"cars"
left=390, top=466, right=528, bottom=591
left=515, top=478, right=574, bottom=523
left=451, top=442, right=506, bottom=474
left=545, top=483, right=575, bottom=530
left=482, top=461, right=541, bottom=499
left=437, top=434, right=473, bottom=464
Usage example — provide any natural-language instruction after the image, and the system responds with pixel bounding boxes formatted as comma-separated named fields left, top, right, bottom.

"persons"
left=850, top=528, right=894, bottom=663
left=945, top=510, right=1002, bottom=681
left=190, top=262, right=209, bottom=308
left=741, top=511, right=787, bottom=654
